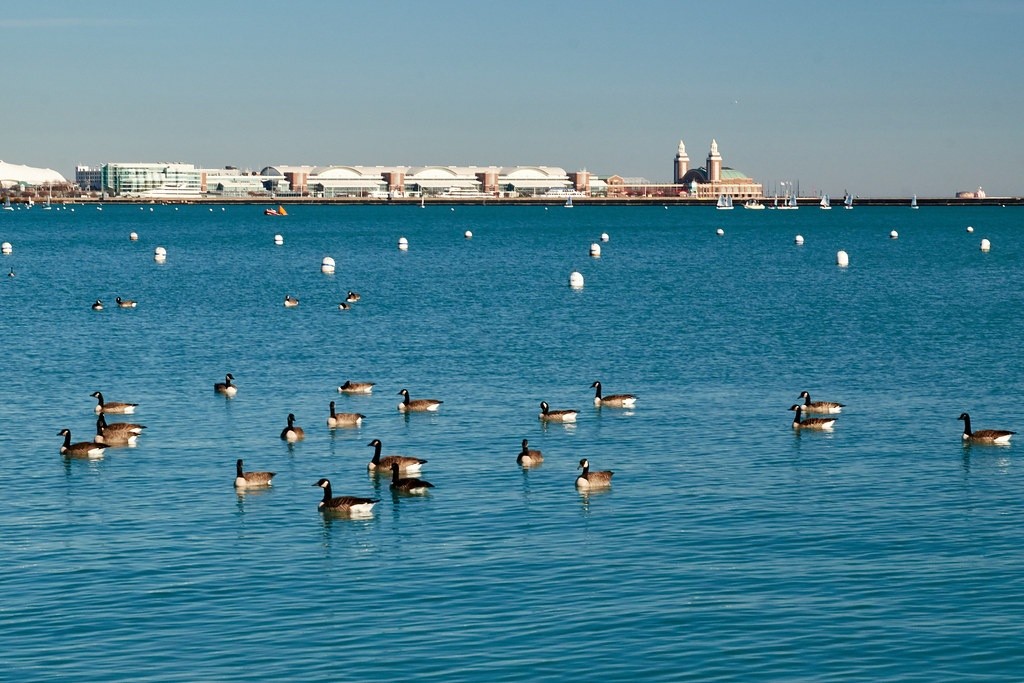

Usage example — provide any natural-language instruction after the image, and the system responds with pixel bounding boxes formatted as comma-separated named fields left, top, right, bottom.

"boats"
left=744, top=199, right=766, bottom=210
left=265, top=205, right=287, bottom=216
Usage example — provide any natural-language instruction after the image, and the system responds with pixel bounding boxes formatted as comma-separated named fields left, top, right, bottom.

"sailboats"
left=3, top=197, right=14, bottom=212
left=43, top=195, right=52, bottom=210
left=768, top=191, right=799, bottom=210
left=844, top=194, right=854, bottom=209
left=819, top=193, right=831, bottom=210
left=911, top=194, right=919, bottom=210
left=563, top=196, right=574, bottom=208
left=715, top=192, right=734, bottom=210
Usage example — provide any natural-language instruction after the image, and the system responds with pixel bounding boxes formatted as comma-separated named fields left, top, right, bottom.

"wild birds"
left=337, top=380, right=377, bottom=393
left=213, top=373, right=238, bottom=395
left=787, top=404, right=839, bottom=429
left=326, top=400, right=366, bottom=429
left=396, top=388, right=445, bottom=412
left=234, top=459, right=277, bottom=487
left=92, top=299, right=104, bottom=310
left=284, top=294, right=299, bottom=307
left=56, top=429, right=112, bottom=458
left=957, top=412, right=1018, bottom=444
left=8, top=265, right=16, bottom=276
left=310, top=477, right=382, bottom=514
left=115, top=297, right=138, bottom=308
left=516, top=438, right=544, bottom=467
left=339, top=303, right=350, bottom=310
left=574, top=458, right=616, bottom=490
left=92, top=411, right=147, bottom=444
left=279, top=413, right=304, bottom=442
left=346, top=291, right=361, bottom=303
left=89, top=390, right=140, bottom=414
left=538, top=400, right=581, bottom=423
left=367, top=438, right=428, bottom=473
left=796, top=390, right=847, bottom=415
left=588, top=380, right=641, bottom=407
left=388, top=462, right=436, bottom=495
